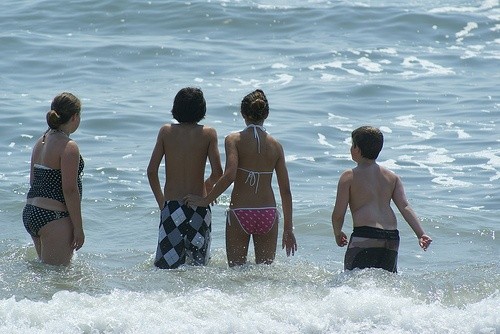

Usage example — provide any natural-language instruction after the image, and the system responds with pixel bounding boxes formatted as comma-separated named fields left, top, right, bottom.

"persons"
left=147, top=87, right=223, bottom=269
left=332, top=126, right=432, bottom=273
left=22, top=92, right=85, bottom=266
left=183, top=89, right=297, bottom=268
